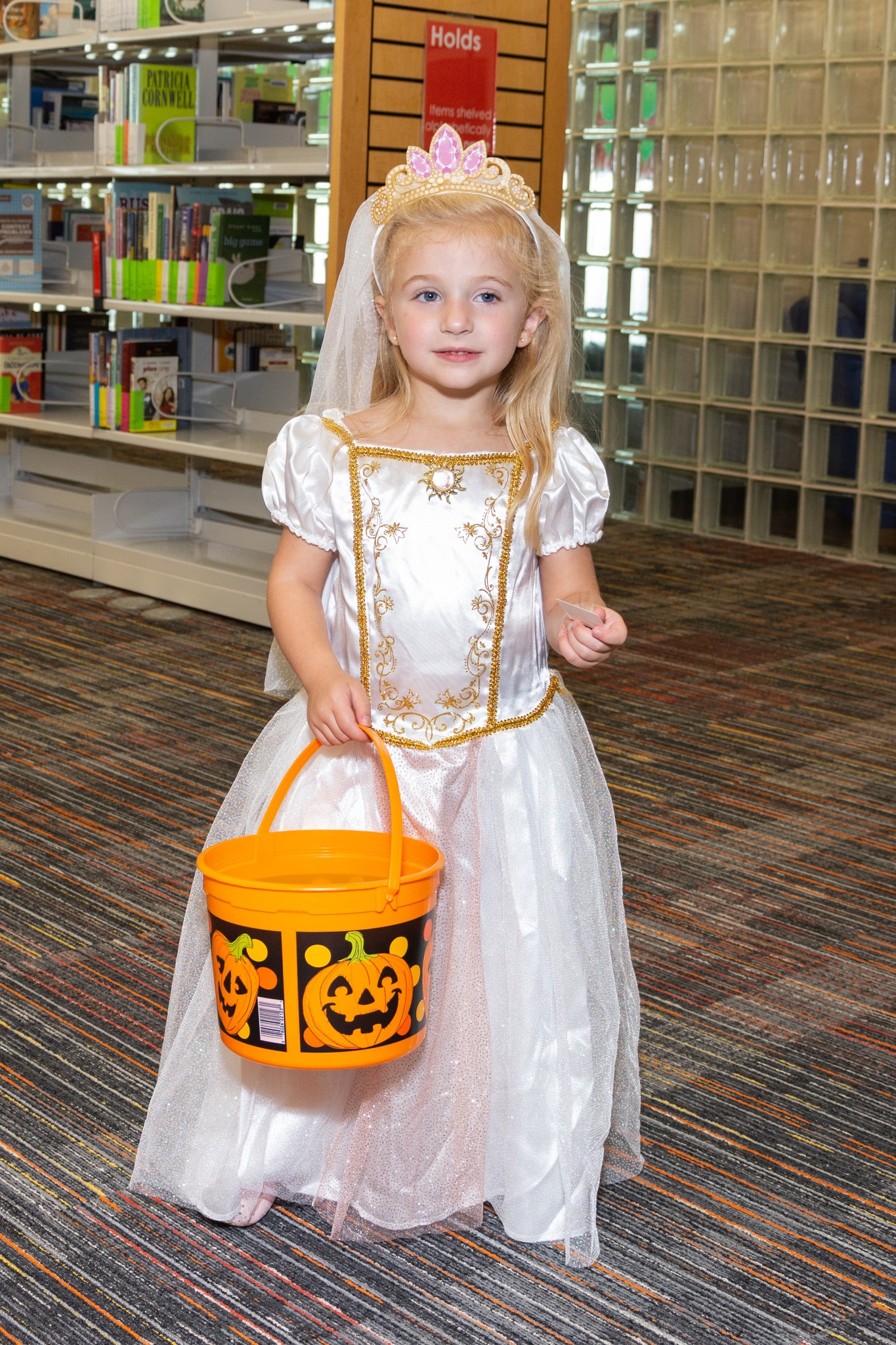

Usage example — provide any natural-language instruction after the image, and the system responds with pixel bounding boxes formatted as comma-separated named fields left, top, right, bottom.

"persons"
left=127, top=123, right=650, bottom=1269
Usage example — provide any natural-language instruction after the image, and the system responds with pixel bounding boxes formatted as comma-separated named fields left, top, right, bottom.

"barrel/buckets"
left=195, top=724, right=445, bottom=1071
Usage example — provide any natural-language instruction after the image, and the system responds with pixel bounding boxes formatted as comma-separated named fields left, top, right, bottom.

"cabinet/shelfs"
left=0, top=0, right=345, bottom=627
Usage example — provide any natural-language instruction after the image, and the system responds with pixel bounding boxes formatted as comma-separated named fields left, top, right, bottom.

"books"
left=0, top=0, right=317, bottom=435
